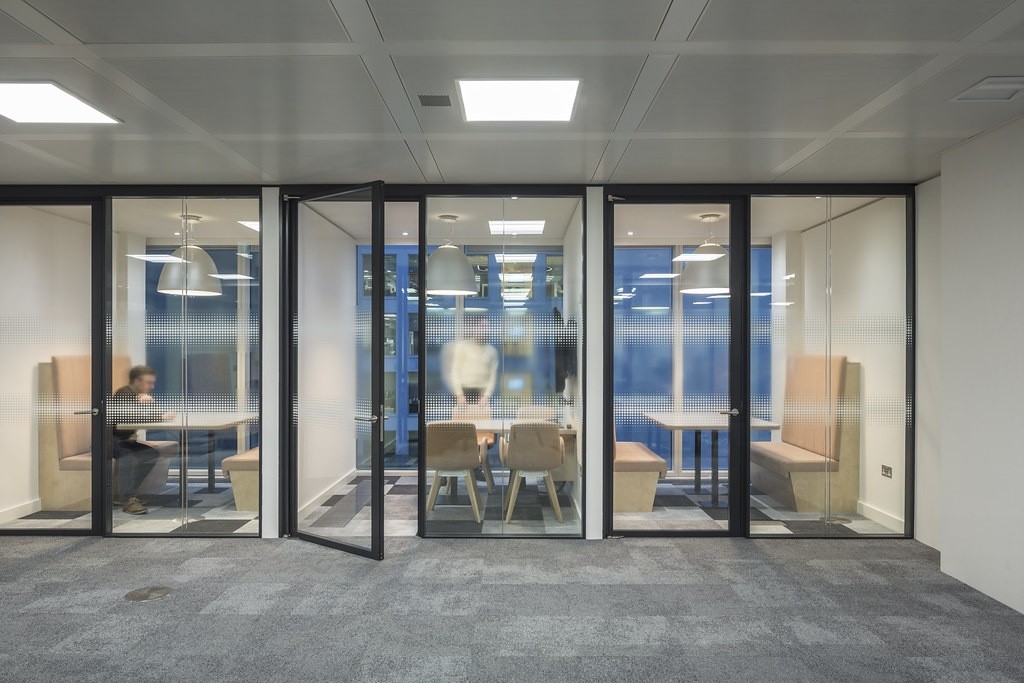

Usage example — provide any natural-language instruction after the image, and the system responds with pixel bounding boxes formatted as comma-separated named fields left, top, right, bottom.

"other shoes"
left=123, top=498, right=148, bottom=514
left=114, top=497, right=147, bottom=506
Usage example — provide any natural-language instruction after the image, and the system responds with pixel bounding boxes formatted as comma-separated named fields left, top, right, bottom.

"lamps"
left=426, top=215, right=478, bottom=296
left=157, top=215, right=223, bottom=297
left=679, top=213, right=729, bottom=295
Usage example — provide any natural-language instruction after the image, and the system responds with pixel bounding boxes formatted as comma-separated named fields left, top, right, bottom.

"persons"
left=109, top=366, right=176, bottom=514
left=440, top=320, right=498, bottom=408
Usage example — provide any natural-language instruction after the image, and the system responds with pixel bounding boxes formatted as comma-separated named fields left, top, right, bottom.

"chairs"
left=425, top=404, right=565, bottom=523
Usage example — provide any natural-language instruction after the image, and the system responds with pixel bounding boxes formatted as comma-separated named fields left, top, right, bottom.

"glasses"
left=136, top=377, right=155, bottom=384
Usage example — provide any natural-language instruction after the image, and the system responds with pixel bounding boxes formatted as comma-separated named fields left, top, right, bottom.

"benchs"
left=38, top=353, right=180, bottom=512
left=613, top=422, right=668, bottom=514
left=221, top=445, right=260, bottom=511
left=749, top=355, right=863, bottom=514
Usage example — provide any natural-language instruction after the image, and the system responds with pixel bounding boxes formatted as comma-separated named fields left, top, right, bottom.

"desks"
left=645, top=412, right=781, bottom=509
left=435, top=418, right=577, bottom=507
left=116, top=410, right=259, bottom=508
